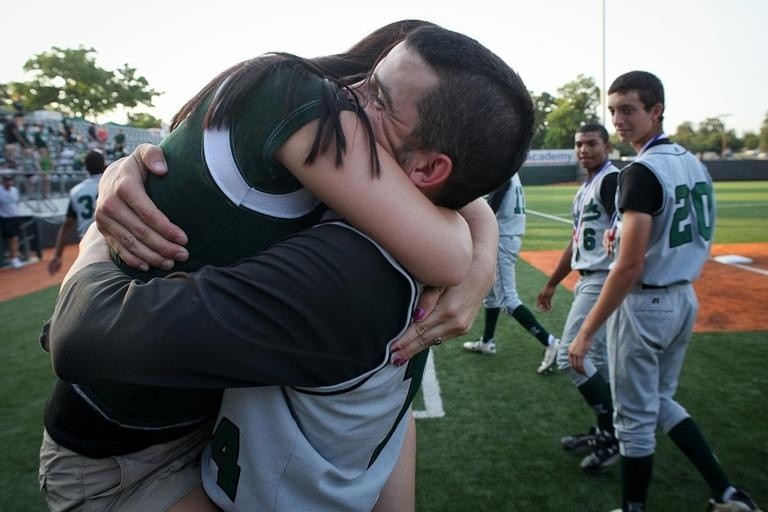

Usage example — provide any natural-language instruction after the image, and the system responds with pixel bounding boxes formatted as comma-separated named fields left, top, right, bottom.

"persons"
left=539, top=124, right=622, bottom=474
left=0, top=109, right=128, bottom=279
left=462, top=171, right=560, bottom=375
left=566, top=71, right=766, bottom=512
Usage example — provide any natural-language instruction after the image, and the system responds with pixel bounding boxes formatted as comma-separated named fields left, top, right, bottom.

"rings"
left=434, top=338, right=444, bottom=347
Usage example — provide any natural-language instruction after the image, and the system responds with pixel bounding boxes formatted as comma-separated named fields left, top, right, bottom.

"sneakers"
left=462, top=338, right=496, bottom=355
left=580, top=444, right=620, bottom=473
left=561, top=426, right=611, bottom=448
left=709, top=489, right=762, bottom=512
left=538, top=338, right=560, bottom=375
left=11, top=258, right=22, bottom=267
